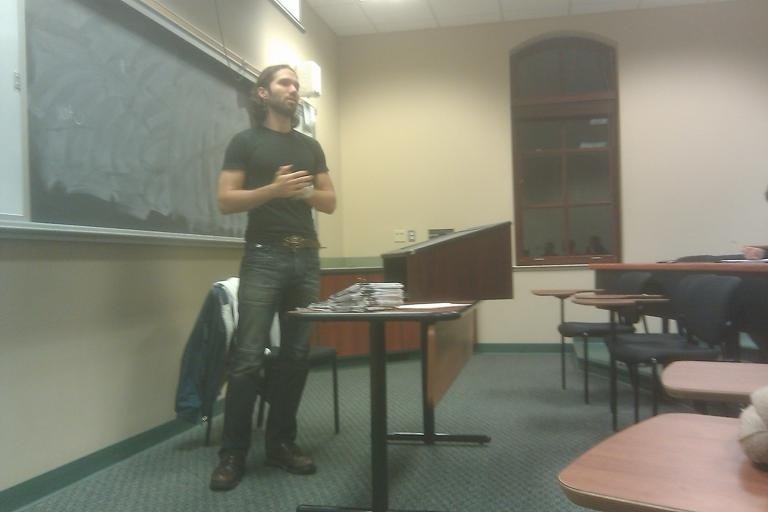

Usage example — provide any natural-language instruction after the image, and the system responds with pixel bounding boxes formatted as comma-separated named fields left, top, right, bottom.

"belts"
left=261, top=232, right=320, bottom=249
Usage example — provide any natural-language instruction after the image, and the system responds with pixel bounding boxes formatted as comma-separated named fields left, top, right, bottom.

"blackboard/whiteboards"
left=0, top=2, right=325, bottom=251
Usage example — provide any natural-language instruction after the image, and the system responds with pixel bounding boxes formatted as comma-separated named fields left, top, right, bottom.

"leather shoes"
left=264, top=441, right=314, bottom=474
left=209, top=453, right=246, bottom=491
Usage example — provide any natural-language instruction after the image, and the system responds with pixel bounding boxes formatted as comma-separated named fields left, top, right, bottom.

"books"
left=328, top=282, right=404, bottom=312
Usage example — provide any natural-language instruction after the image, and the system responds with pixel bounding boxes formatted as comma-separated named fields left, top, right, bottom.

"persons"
left=210, top=64, right=337, bottom=490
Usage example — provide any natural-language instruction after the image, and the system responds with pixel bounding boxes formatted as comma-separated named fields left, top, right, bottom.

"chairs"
left=202, top=276, right=343, bottom=449
left=531, top=270, right=742, bottom=429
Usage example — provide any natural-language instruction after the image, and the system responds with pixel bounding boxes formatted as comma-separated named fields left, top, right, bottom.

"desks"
left=560, top=347, right=768, bottom=512
left=584, top=260, right=767, bottom=337
left=288, top=295, right=496, bottom=511
